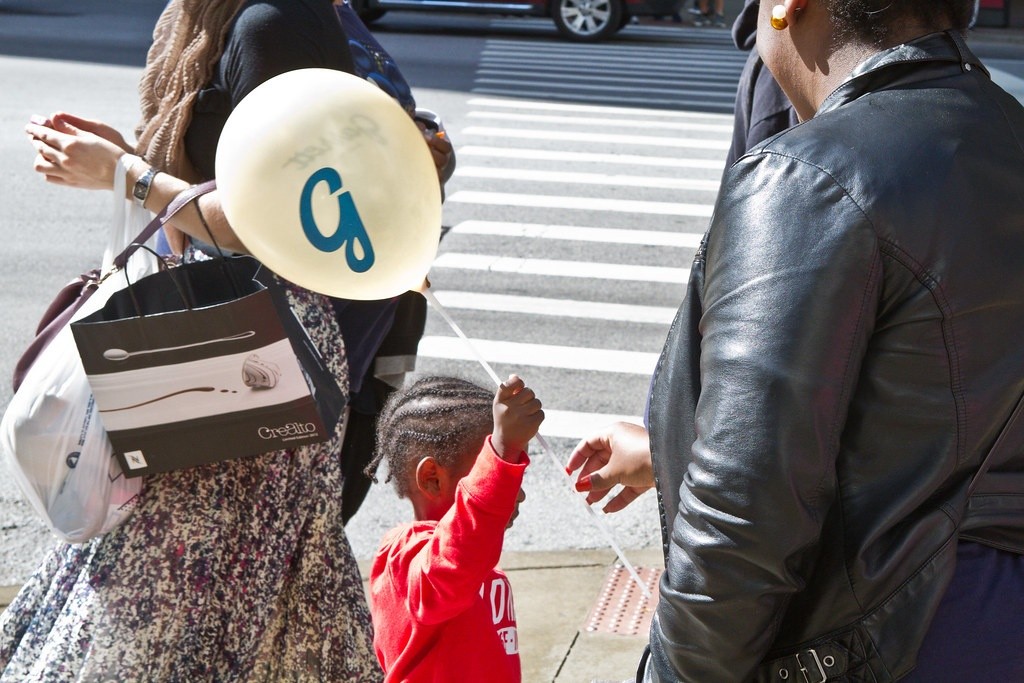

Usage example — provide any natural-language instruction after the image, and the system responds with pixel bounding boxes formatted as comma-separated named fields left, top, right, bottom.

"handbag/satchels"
left=5, top=199, right=346, bottom=547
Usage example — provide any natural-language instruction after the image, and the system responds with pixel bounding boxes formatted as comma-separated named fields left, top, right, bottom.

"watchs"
left=132, top=165, right=163, bottom=209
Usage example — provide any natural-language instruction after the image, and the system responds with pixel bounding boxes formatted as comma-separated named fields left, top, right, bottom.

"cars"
left=341, top=0, right=633, bottom=41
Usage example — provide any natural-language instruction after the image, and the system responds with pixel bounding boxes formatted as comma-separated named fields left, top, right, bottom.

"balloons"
left=214, top=67, right=443, bottom=301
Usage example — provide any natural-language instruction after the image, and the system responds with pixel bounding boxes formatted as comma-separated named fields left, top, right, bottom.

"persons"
left=6, top=0, right=384, bottom=680
left=324, top=0, right=456, bottom=529
left=654, top=0, right=727, bottom=28
left=721, top=1, right=803, bottom=173
left=565, top=0, right=1024, bottom=681
left=364, top=373, right=546, bottom=683
